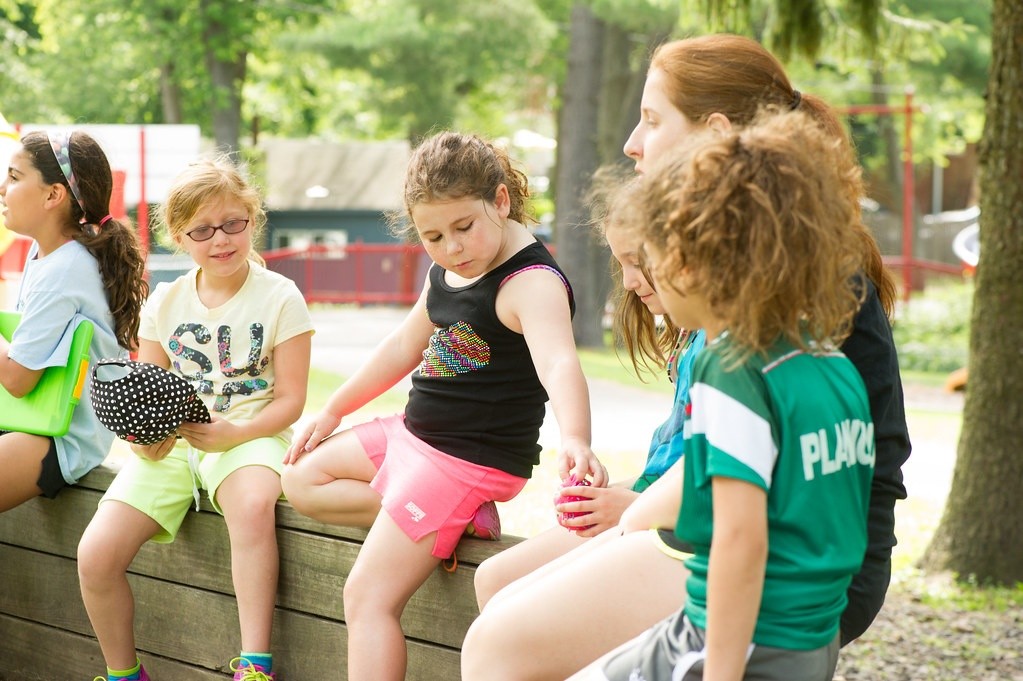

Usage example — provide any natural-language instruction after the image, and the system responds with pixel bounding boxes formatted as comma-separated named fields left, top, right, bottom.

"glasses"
left=183, top=209, right=251, bottom=242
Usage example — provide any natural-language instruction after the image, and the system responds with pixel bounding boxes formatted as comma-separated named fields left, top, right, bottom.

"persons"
left=0, top=130, right=149, bottom=512
left=460, top=34, right=912, bottom=681
left=76, top=162, right=315, bottom=681
left=282, top=134, right=609, bottom=680
left=564, top=111, right=876, bottom=681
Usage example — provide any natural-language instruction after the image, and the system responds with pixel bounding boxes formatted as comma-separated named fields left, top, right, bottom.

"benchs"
left=0, top=457, right=558, bottom=680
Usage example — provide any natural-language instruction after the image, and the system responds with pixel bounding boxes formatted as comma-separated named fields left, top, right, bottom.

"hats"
left=89, top=357, right=211, bottom=444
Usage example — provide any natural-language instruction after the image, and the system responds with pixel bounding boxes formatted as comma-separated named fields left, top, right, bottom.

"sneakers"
left=229, top=656, right=276, bottom=681
left=94, top=663, right=151, bottom=681
